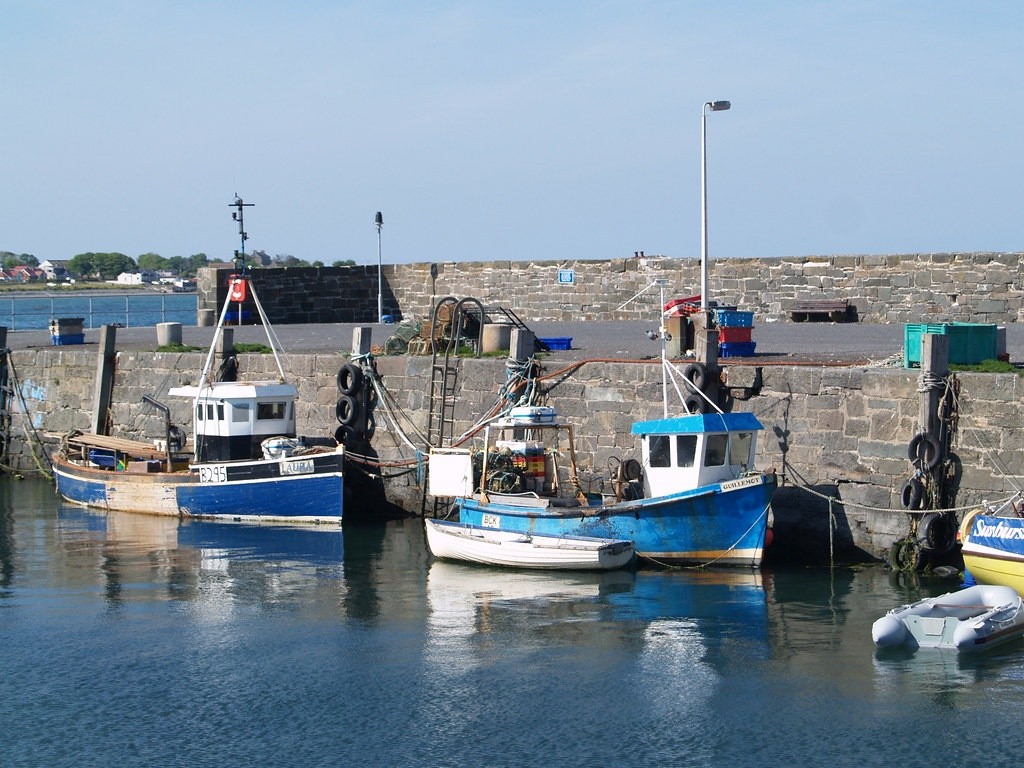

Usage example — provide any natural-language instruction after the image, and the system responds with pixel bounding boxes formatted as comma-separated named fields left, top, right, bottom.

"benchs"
left=788, top=301, right=848, bottom=322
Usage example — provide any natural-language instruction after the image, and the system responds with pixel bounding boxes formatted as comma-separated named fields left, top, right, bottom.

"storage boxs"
left=721, top=343, right=756, bottom=357
left=526, top=477, right=544, bottom=492
left=48, top=317, right=86, bottom=345
left=717, top=309, right=754, bottom=327
left=539, top=338, right=574, bottom=350
left=511, top=456, right=545, bottom=476
left=720, top=326, right=754, bottom=342
left=496, top=440, right=545, bottom=455
left=512, top=406, right=557, bottom=422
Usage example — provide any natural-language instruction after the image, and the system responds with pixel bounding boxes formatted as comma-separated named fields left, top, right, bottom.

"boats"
left=958, top=489, right=1024, bottom=597
left=454, top=282, right=778, bottom=572
left=51, top=191, right=348, bottom=527
left=872, top=583, right=1024, bottom=656
left=51, top=497, right=346, bottom=583
left=424, top=518, right=637, bottom=574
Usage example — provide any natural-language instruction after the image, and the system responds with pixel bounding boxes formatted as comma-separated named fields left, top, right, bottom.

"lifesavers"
left=683, top=362, right=709, bottom=395
left=959, top=508, right=984, bottom=542
left=336, top=395, right=359, bottom=426
left=683, top=394, right=708, bottom=414
left=908, top=432, right=943, bottom=471
left=335, top=425, right=355, bottom=446
left=900, top=478, right=922, bottom=514
left=916, top=512, right=953, bottom=551
left=337, top=363, right=362, bottom=395
left=890, top=535, right=926, bottom=571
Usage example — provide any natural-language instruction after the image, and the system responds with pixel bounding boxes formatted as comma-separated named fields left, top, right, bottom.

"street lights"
left=374, top=211, right=385, bottom=322
left=700, top=100, right=732, bottom=315
